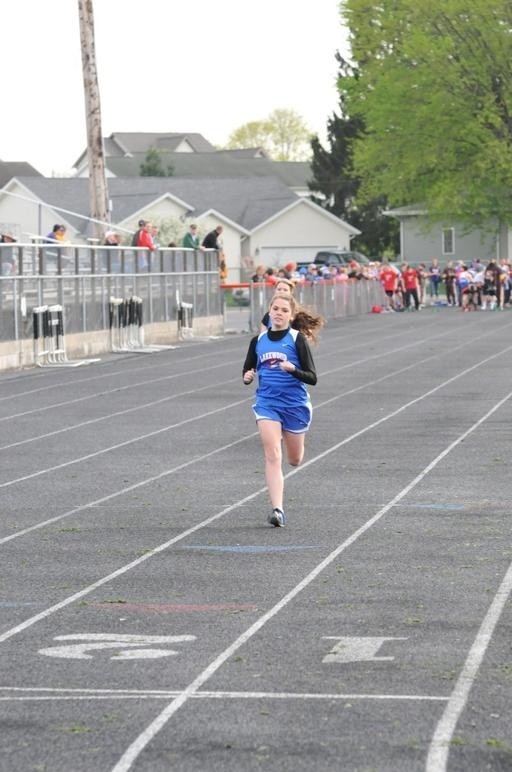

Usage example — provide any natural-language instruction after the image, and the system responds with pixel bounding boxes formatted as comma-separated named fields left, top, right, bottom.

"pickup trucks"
left=297, top=251, right=371, bottom=270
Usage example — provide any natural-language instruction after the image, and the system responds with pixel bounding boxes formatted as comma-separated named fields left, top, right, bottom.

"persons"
left=251, top=259, right=512, bottom=311
left=42, top=224, right=67, bottom=244
left=104, top=231, right=118, bottom=246
left=183, top=224, right=206, bottom=252
left=132, top=219, right=160, bottom=250
left=202, top=224, right=223, bottom=249
left=242, top=295, right=327, bottom=528
left=259, top=279, right=295, bottom=335
left=0, top=229, right=18, bottom=277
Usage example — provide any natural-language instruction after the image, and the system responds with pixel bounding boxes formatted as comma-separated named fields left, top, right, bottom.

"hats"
left=102, top=229, right=123, bottom=245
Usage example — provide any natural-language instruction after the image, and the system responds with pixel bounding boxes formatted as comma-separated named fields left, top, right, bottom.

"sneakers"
left=268, top=509, right=286, bottom=529
